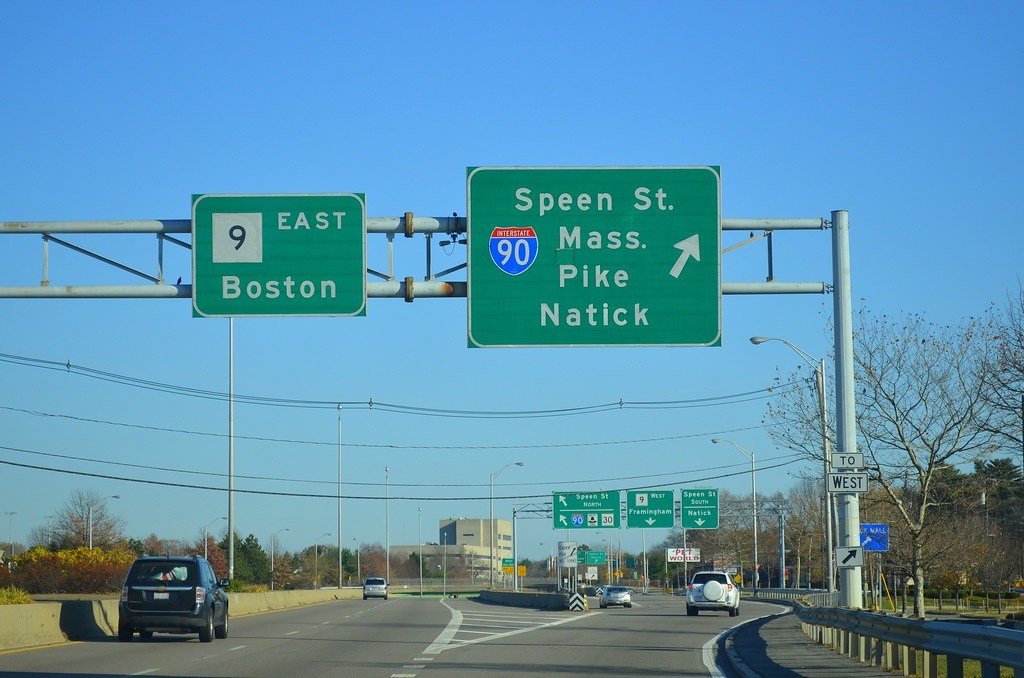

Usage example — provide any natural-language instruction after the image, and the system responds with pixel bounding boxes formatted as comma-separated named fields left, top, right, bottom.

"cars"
left=599, top=586, right=632, bottom=608
left=363, top=577, right=390, bottom=600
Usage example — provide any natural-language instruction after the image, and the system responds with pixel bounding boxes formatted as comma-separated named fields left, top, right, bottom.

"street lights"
left=315, top=533, right=331, bottom=584
left=443, top=532, right=447, bottom=596
left=710, top=438, right=758, bottom=589
left=205, top=517, right=228, bottom=560
left=337, top=402, right=343, bottom=589
left=4, top=511, right=16, bottom=543
left=418, top=506, right=423, bottom=597
left=748, top=336, right=834, bottom=594
left=489, top=461, right=524, bottom=586
left=43, top=515, right=54, bottom=550
left=271, top=528, right=290, bottom=590
left=385, top=466, right=390, bottom=586
left=89, top=495, right=121, bottom=550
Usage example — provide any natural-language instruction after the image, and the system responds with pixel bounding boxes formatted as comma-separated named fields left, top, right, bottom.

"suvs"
left=117, top=556, right=231, bottom=643
left=686, top=570, right=743, bottom=617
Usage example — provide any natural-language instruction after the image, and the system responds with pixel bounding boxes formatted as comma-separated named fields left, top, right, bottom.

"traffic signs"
left=558, top=540, right=577, bottom=567
left=859, top=523, right=890, bottom=551
left=465, top=166, right=722, bottom=347
left=682, top=487, right=719, bottom=528
left=626, top=490, right=675, bottom=528
left=552, top=490, right=621, bottom=529
